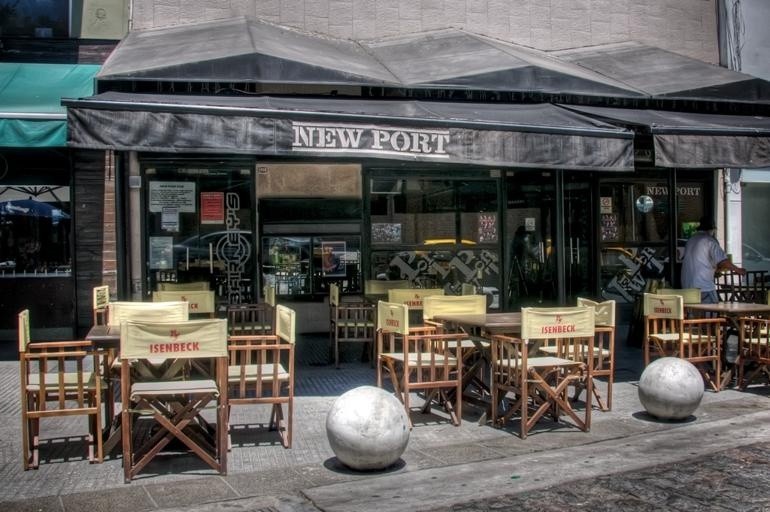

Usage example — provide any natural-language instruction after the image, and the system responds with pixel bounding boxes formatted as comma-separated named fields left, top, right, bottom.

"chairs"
left=158, top=281, right=211, bottom=290
left=625, top=278, right=769, bottom=398
left=329, top=282, right=377, bottom=369
left=92, top=284, right=112, bottom=371
left=488, top=304, right=597, bottom=439
left=114, top=315, right=234, bottom=487
left=364, top=278, right=412, bottom=292
left=103, top=295, right=193, bottom=439
left=388, top=288, right=441, bottom=310
left=536, top=296, right=618, bottom=413
left=14, top=305, right=113, bottom=469
left=421, top=292, right=488, bottom=351
left=458, top=281, right=478, bottom=297
left=372, top=298, right=470, bottom=426
left=224, top=300, right=298, bottom=453
left=225, top=285, right=279, bottom=348
left=152, top=288, right=216, bottom=378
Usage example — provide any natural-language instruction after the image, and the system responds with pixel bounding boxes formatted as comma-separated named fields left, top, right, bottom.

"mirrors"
left=634, top=194, right=655, bottom=214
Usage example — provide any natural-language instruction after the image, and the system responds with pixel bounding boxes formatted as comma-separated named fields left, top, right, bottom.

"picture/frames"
left=321, top=240, right=347, bottom=279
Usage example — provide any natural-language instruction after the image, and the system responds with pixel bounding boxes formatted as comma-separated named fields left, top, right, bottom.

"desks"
left=430, top=311, right=526, bottom=427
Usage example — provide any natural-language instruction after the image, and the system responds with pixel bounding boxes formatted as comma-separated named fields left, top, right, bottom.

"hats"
left=696, top=218, right=716, bottom=229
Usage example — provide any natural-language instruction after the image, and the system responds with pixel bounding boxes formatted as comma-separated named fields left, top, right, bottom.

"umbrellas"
left=0, top=196, right=71, bottom=240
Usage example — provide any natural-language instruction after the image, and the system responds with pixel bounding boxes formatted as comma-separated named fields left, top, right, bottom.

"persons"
left=681, top=215, right=745, bottom=373
left=509, top=226, right=541, bottom=295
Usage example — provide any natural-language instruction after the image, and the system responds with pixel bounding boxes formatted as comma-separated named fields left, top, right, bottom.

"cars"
left=165, top=231, right=315, bottom=275
left=541, top=236, right=649, bottom=273
left=405, top=239, right=478, bottom=267
